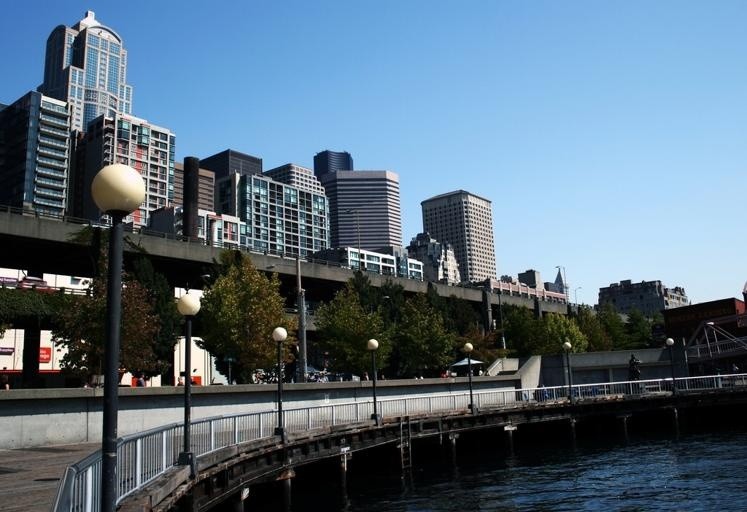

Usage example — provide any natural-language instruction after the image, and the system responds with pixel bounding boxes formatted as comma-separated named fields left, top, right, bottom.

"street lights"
left=91, top=164, right=147, bottom=512
left=465, top=343, right=477, bottom=409
left=273, top=327, right=288, bottom=434
left=177, top=294, right=201, bottom=463
left=667, top=338, right=677, bottom=395
left=368, top=339, right=381, bottom=419
left=563, top=342, right=574, bottom=399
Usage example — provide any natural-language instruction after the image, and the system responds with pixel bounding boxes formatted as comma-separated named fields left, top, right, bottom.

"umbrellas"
left=451, top=356, right=485, bottom=366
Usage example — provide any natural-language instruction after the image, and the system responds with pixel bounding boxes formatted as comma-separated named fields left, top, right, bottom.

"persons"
left=176, top=376, right=183, bottom=386
left=136, top=371, right=145, bottom=387
left=627, top=353, right=640, bottom=380
